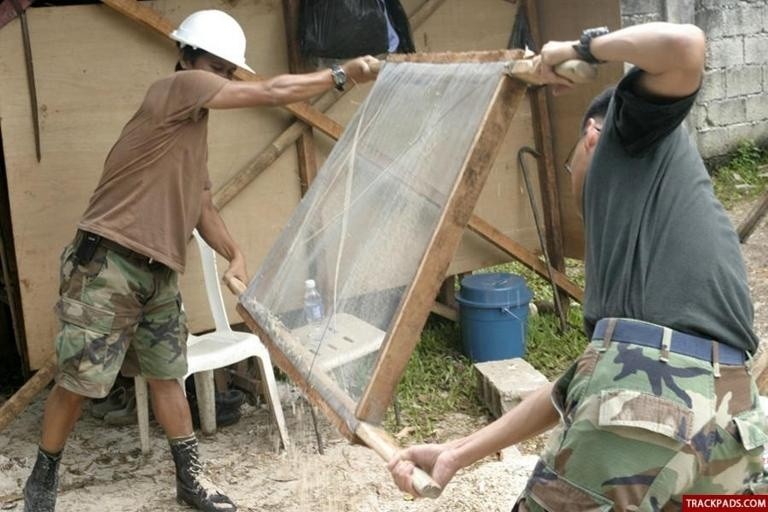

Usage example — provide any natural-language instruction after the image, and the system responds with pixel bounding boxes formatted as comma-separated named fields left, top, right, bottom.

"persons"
left=386, top=21, right=764, bottom=511
left=23, top=10, right=382, bottom=511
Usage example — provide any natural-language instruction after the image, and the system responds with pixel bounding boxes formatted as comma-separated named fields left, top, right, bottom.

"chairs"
left=133, top=226, right=293, bottom=458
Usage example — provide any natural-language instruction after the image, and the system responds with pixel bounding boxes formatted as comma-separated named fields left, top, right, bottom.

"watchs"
left=330, top=64, right=347, bottom=88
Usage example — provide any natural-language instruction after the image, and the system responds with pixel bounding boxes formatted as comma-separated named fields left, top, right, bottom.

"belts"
left=590, top=319, right=745, bottom=366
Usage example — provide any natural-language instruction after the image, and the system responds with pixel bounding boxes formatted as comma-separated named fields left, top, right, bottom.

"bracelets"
left=574, top=26, right=611, bottom=64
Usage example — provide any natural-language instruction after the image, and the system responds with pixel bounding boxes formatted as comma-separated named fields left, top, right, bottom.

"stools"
left=286, top=312, right=408, bottom=455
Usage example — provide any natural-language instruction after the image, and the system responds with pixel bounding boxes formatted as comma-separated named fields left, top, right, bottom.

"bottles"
left=301, top=280, right=326, bottom=343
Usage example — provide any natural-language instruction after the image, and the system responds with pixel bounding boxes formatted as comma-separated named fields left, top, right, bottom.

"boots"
left=24, top=447, right=62, bottom=512
left=170, top=438, right=235, bottom=512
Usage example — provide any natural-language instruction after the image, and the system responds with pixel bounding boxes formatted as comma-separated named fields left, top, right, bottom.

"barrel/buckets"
left=452, top=270, right=535, bottom=363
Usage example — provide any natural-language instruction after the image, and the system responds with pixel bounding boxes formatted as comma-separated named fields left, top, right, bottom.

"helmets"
left=168, top=10, right=256, bottom=75
